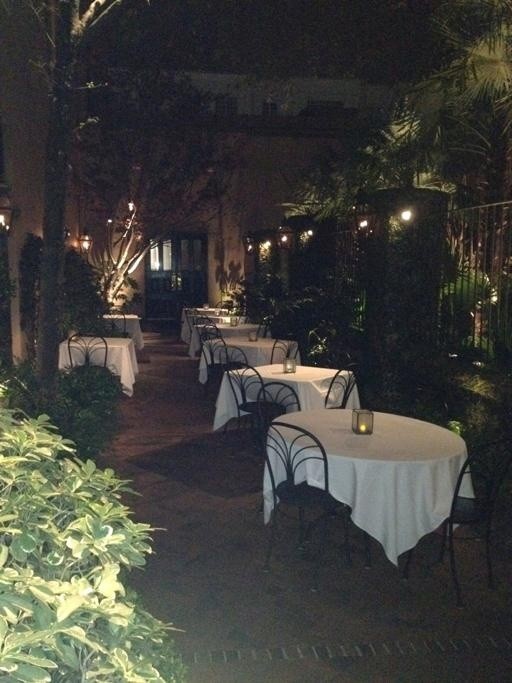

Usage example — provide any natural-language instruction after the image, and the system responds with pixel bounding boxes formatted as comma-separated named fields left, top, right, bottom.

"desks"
left=58, top=337, right=139, bottom=398
left=212, top=362, right=363, bottom=444
left=97, top=314, right=144, bottom=351
left=262, top=407, right=477, bottom=573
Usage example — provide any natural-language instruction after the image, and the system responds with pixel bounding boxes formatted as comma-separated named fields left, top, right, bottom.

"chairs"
left=186, top=299, right=302, bottom=391
left=402, top=438, right=509, bottom=609
left=261, top=422, right=369, bottom=573
left=225, top=360, right=268, bottom=442
left=100, top=309, right=126, bottom=336
left=68, top=332, right=108, bottom=367
left=325, top=361, right=362, bottom=408
left=256, top=383, right=302, bottom=445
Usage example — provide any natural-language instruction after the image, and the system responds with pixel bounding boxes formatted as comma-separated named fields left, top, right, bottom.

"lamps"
left=79, top=227, right=92, bottom=254
left=0, top=191, right=20, bottom=235
